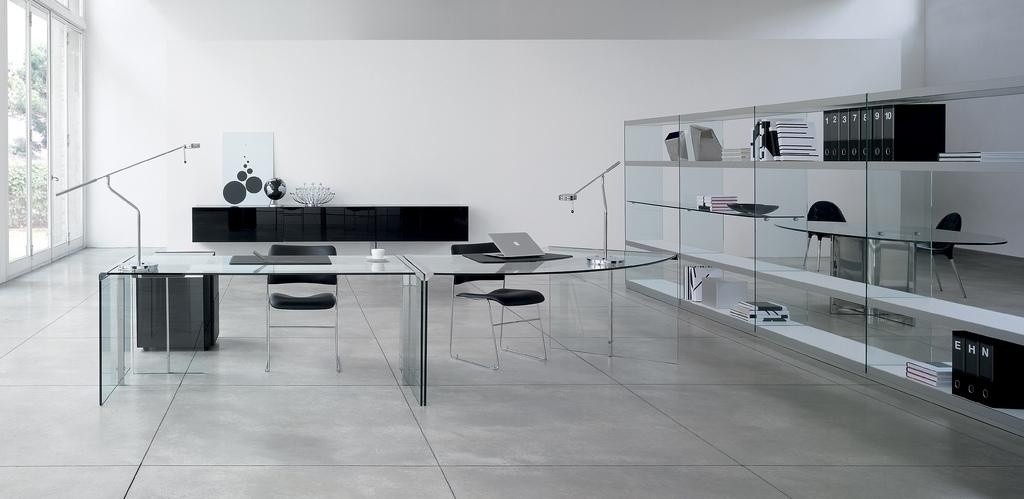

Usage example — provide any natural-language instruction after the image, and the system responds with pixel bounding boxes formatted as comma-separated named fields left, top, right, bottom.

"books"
left=938, top=152, right=1024, bottom=157
left=905, top=361, right=953, bottom=386
left=682, top=114, right=821, bottom=324
left=938, top=156, right=1024, bottom=162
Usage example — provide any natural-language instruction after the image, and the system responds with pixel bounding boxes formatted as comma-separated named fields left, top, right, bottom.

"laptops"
left=483, top=232, right=546, bottom=259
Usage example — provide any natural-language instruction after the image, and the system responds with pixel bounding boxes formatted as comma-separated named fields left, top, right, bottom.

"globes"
left=264, top=177, right=288, bottom=207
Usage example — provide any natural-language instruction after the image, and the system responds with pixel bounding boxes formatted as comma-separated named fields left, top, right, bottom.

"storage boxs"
left=702, top=276, right=748, bottom=309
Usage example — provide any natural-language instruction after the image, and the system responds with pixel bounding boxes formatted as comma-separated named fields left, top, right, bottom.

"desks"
left=774, top=220, right=1008, bottom=326
left=402, top=245, right=682, bottom=405
left=98, top=255, right=423, bottom=406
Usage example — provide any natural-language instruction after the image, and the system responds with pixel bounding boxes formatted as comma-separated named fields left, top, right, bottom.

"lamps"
left=55, top=142, right=202, bottom=270
left=558, top=161, right=626, bottom=265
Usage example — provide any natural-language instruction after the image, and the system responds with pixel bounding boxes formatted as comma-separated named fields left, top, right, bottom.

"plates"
left=366, top=255, right=389, bottom=262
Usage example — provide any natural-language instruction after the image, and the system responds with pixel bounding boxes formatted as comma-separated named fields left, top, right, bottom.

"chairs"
left=913, top=212, right=969, bottom=300
left=264, top=244, right=341, bottom=375
left=449, top=241, right=548, bottom=371
left=802, top=200, right=848, bottom=272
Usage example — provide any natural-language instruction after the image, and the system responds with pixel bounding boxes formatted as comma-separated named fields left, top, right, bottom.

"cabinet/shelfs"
left=190, top=205, right=470, bottom=256
left=133, top=250, right=220, bottom=353
left=621, top=77, right=1023, bottom=440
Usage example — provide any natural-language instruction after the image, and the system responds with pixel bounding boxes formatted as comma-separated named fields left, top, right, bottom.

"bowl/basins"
left=370, top=248, right=384, bottom=258
